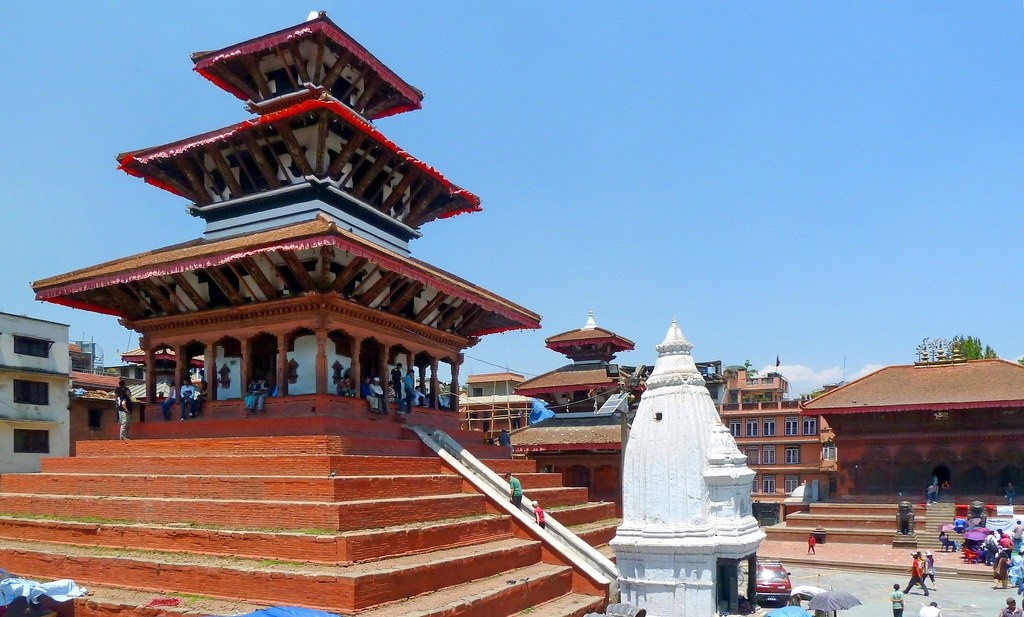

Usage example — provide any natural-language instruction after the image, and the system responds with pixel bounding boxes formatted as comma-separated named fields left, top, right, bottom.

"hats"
left=374, top=377, right=380, bottom=382
left=506, top=471, right=511, bottom=475
left=925, top=550, right=931, bottom=555
left=1006, top=597, right=1016, bottom=603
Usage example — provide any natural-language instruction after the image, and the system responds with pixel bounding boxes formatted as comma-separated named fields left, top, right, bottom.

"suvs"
left=756, top=560, right=792, bottom=603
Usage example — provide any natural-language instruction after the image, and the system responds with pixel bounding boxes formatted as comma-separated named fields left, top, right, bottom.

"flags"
left=776, top=354, right=780, bottom=366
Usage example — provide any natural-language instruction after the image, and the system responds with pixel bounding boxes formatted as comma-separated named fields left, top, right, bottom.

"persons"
left=927, top=480, right=953, bottom=503
left=115, top=367, right=269, bottom=438
left=498, top=428, right=510, bottom=446
left=336, top=363, right=450, bottom=415
left=939, top=521, right=1024, bottom=617
left=588, top=377, right=646, bottom=403
left=1006, top=483, right=1014, bottom=505
left=506, top=472, right=523, bottom=511
left=898, top=489, right=903, bottom=496
left=532, top=501, right=545, bottom=529
left=920, top=602, right=940, bottom=617
left=891, top=584, right=904, bottom=617
left=903, top=550, right=937, bottom=596
left=738, top=595, right=755, bottom=615
left=808, top=534, right=815, bottom=555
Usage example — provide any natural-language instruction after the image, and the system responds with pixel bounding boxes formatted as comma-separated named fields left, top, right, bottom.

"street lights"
left=597, top=364, right=647, bottom=465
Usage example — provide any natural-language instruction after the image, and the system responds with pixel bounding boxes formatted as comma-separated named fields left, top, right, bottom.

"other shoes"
left=248, top=410, right=253, bottom=414
left=372, top=409, right=408, bottom=415
left=181, top=418, right=184, bottom=421
left=259, top=410, right=263, bottom=414
left=189, top=413, right=193, bottom=416
left=123, top=437, right=130, bottom=441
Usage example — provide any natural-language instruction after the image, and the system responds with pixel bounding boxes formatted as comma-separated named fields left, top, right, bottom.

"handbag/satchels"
left=986, top=544, right=998, bottom=553
left=183, top=390, right=191, bottom=399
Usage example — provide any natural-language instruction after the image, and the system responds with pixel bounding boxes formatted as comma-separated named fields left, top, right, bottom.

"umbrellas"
left=935, top=523, right=955, bottom=532
left=763, top=586, right=863, bottom=617
left=963, top=518, right=991, bottom=541
